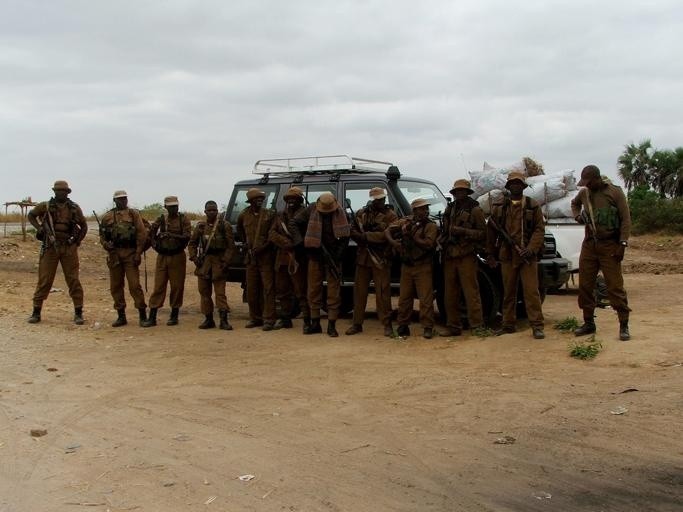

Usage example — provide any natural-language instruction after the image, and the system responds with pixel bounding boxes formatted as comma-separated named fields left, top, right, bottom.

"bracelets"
left=621, top=242, right=626, bottom=246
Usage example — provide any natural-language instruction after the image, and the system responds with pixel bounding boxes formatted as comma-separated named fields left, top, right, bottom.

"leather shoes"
left=263, top=322, right=273, bottom=331
left=274, top=320, right=291, bottom=329
left=397, top=325, right=410, bottom=335
left=493, top=326, right=515, bottom=334
left=533, top=329, right=544, bottom=339
left=423, top=327, right=432, bottom=337
left=619, top=321, right=629, bottom=340
left=302, top=317, right=310, bottom=332
left=345, top=324, right=363, bottom=334
left=574, top=317, right=595, bottom=335
left=440, top=328, right=461, bottom=336
left=246, top=320, right=260, bottom=327
left=384, top=326, right=392, bottom=335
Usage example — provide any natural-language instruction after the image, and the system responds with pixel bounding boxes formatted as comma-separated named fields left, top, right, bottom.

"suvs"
left=216, top=156, right=572, bottom=322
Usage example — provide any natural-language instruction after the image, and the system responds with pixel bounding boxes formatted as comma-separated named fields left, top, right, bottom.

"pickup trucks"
left=409, top=194, right=586, bottom=294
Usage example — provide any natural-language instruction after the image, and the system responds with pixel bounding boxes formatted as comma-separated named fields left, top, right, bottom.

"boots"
left=198, top=313, right=215, bottom=328
left=138, top=308, right=146, bottom=324
left=145, top=309, right=157, bottom=326
left=308, top=318, right=322, bottom=333
left=29, top=307, right=41, bottom=323
left=166, top=308, right=179, bottom=325
left=74, top=307, right=83, bottom=324
left=327, top=320, right=339, bottom=336
left=219, top=313, right=232, bottom=329
left=112, top=309, right=127, bottom=326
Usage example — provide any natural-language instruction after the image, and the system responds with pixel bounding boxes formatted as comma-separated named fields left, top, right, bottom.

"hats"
left=284, top=187, right=304, bottom=201
left=449, top=180, right=474, bottom=195
left=370, top=187, right=386, bottom=200
left=113, top=191, right=127, bottom=199
left=577, top=165, right=599, bottom=186
left=165, top=196, right=179, bottom=206
left=411, top=198, right=431, bottom=209
left=505, top=172, right=529, bottom=187
left=245, top=188, right=267, bottom=202
left=52, top=181, right=71, bottom=193
left=316, top=193, right=340, bottom=212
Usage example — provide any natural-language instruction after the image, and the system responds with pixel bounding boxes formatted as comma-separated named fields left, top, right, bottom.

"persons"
left=287, top=191, right=352, bottom=338
left=482, top=171, right=546, bottom=339
left=188, top=200, right=236, bottom=331
left=434, top=179, right=496, bottom=337
left=268, top=187, right=314, bottom=335
left=27, top=180, right=88, bottom=324
left=96, top=189, right=147, bottom=328
left=383, top=197, right=440, bottom=339
left=234, top=188, right=278, bottom=331
left=346, top=187, right=399, bottom=339
left=142, top=194, right=190, bottom=327
left=569, top=164, right=633, bottom=341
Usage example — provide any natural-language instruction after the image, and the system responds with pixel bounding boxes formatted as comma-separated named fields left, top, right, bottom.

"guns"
left=488, top=212, right=531, bottom=266
left=38, top=209, right=64, bottom=262
left=392, top=219, right=416, bottom=240
left=253, top=199, right=277, bottom=258
left=197, top=212, right=225, bottom=267
left=158, top=213, right=173, bottom=255
left=344, top=195, right=389, bottom=270
left=297, top=219, right=346, bottom=284
left=581, top=207, right=604, bottom=245
left=91, top=210, right=115, bottom=255
left=438, top=198, right=451, bottom=265
